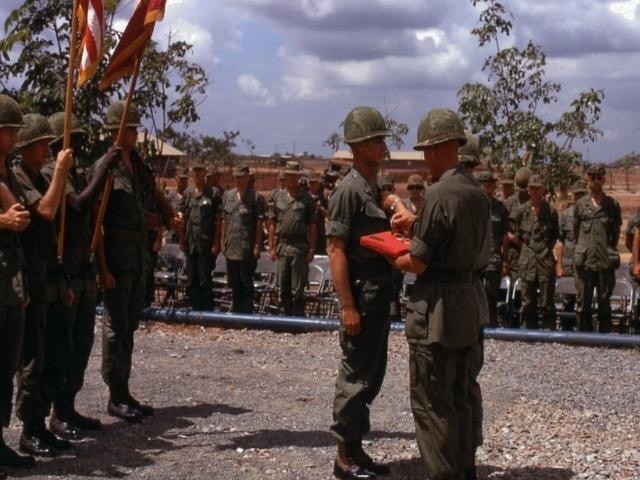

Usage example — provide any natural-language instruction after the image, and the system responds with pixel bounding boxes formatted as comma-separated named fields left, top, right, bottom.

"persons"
left=1, top=95, right=639, bottom=480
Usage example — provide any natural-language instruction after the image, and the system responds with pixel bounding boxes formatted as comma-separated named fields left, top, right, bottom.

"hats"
left=571, top=179, right=587, bottom=193
left=586, top=165, right=606, bottom=175
left=174, top=160, right=303, bottom=182
left=496, top=163, right=517, bottom=185
left=526, top=174, right=546, bottom=188
left=380, top=175, right=396, bottom=188
left=476, top=170, right=499, bottom=182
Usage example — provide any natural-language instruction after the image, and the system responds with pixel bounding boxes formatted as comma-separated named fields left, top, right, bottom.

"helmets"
left=0, top=94, right=27, bottom=129
left=458, top=129, right=482, bottom=166
left=340, top=104, right=393, bottom=145
left=13, top=111, right=57, bottom=150
left=513, top=166, right=534, bottom=192
left=45, top=109, right=93, bottom=148
left=97, top=99, right=143, bottom=130
left=412, top=106, right=467, bottom=152
left=405, top=173, right=424, bottom=190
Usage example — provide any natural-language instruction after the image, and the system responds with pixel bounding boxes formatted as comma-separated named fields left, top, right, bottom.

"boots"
left=333, top=440, right=390, bottom=480
left=0, top=390, right=156, bottom=480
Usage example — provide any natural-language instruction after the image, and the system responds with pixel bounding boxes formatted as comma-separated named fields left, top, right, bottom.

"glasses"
left=590, top=176, right=602, bottom=181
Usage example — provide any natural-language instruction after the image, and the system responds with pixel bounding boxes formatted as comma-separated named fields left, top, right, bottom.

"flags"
left=76, top=0, right=105, bottom=92
left=97, top=0, right=166, bottom=92
